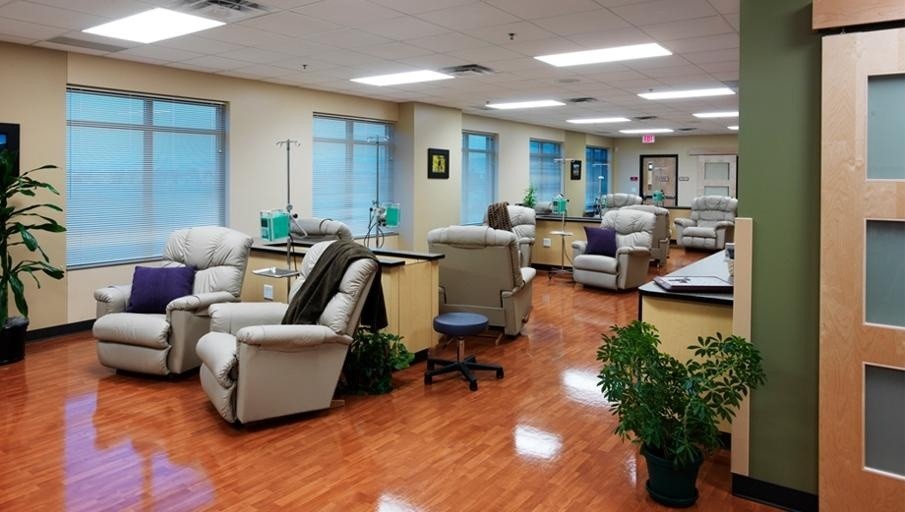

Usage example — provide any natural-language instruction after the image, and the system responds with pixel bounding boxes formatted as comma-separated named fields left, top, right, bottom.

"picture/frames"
left=427, top=147, right=449, bottom=179
left=0, top=122, right=20, bottom=180
left=570, top=160, right=581, bottom=180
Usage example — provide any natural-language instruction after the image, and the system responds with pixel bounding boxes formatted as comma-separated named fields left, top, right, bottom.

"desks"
left=635, top=251, right=735, bottom=451
left=248, top=238, right=446, bottom=369
left=530, top=213, right=602, bottom=271
left=663, top=205, right=693, bottom=242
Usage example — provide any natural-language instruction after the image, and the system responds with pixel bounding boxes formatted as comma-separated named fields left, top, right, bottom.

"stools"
left=421, top=312, right=504, bottom=391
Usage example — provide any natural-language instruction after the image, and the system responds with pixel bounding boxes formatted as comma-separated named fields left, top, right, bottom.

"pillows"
left=583, top=225, right=617, bottom=258
left=124, top=265, right=196, bottom=314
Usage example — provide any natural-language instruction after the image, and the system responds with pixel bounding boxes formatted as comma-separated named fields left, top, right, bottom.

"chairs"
left=92, top=225, right=255, bottom=378
left=620, top=203, right=670, bottom=265
left=194, top=241, right=380, bottom=427
left=424, top=226, right=538, bottom=344
left=571, top=209, right=656, bottom=290
left=483, top=204, right=536, bottom=268
left=673, top=195, right=739, bottom=249
left=593, top=194, right=645, bottom=219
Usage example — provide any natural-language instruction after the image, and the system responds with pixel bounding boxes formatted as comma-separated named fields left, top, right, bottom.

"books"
left=651, top=274, right=733, bottom=294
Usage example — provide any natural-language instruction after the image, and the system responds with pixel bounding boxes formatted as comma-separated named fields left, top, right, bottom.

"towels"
left=272, top=238, right=389, bottom=327
left=487, top=201, right=512, bottom=233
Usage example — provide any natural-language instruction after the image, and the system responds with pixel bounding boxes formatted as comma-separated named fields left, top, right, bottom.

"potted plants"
left=0, top=158, right=66, bottom=364
left=347, top=325, right=415, bottom=396
left=595, top=316, right=769, bottom=508
